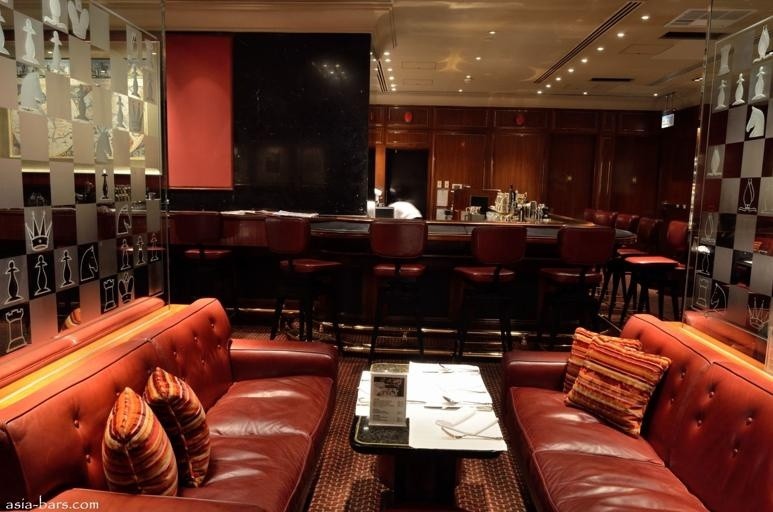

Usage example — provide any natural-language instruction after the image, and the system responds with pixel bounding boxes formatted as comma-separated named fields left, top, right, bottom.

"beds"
left=350, top=361, right=509, bottom=506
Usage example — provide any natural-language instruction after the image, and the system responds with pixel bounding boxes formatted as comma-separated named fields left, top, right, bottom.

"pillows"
left=561, top=326, right=673, bottom=438
left=100, top=367, right=212, bottom=496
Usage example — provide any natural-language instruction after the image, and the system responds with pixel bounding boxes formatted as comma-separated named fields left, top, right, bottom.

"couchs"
left=499, top=312, right=773, bottom=511
left=0, top=298, right=340, bottom=511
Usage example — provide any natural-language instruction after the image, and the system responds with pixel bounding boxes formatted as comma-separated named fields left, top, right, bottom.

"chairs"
left=580, top=208, right=696, bottom=327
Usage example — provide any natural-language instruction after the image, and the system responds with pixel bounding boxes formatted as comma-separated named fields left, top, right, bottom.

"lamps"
left=661, top=94, right=675, bottom=129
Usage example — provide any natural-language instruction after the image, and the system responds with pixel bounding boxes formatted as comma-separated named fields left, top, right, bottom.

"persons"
left=386, top=190, right=422, bottom=219
left=367, top=191, right=376, bottom=219
